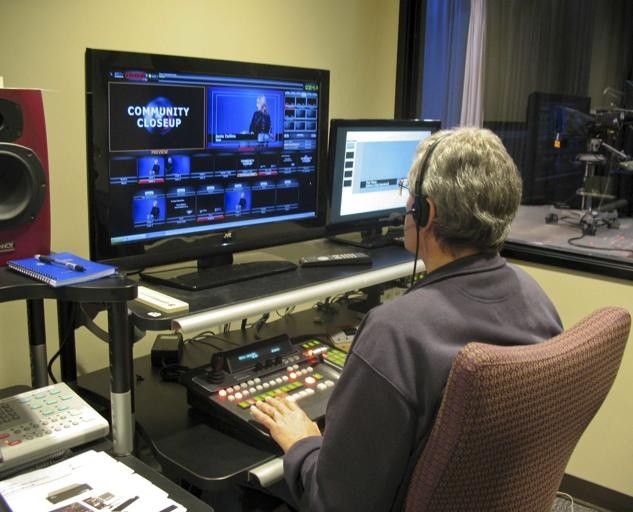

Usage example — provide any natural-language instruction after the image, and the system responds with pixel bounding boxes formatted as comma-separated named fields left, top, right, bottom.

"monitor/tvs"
left=83, top=46, right=330, bottom=291
left=326, top=119, right=440, bottom=249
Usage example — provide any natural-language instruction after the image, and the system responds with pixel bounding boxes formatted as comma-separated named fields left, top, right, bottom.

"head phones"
left=412, top=133, right=448, bottom=225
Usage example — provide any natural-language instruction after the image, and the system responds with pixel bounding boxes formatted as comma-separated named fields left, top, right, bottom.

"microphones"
left=379, top=213, right=406, bottom=226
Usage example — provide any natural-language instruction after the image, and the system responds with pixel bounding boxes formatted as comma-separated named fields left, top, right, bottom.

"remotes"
left=300, top=251, right=376, bottom=267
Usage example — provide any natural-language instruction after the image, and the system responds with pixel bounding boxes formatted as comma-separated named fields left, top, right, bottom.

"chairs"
left=397, top=305, right=632, bottom=511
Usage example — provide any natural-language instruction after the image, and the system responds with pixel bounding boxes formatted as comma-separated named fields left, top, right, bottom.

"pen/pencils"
left=34, top=254, right=86, bottom=272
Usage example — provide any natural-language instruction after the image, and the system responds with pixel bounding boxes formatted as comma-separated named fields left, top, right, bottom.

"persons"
left=152, top=159, right=160, bottom=176
left=238, top=192, right=247, bottom=209
left=250, top=126, right=564, bottom=512
left=151, top=200, right=159, bottom=220
left=249, top=95, right=272, bottom=135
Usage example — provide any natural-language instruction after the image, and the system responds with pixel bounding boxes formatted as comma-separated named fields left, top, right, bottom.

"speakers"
left=0, top=87, right=51, bottom=267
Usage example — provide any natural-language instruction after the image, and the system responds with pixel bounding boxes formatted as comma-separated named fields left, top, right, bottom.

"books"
left=8, top=251, right=115, bottom=288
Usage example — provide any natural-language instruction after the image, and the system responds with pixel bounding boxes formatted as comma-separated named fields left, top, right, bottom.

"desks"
left=0, top=262, right=214, bottom=511
left=55, top=232, right=426, bottom=511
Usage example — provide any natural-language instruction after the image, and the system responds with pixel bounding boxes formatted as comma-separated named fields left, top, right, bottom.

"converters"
left=151, top=333, right=184, bottom=368
